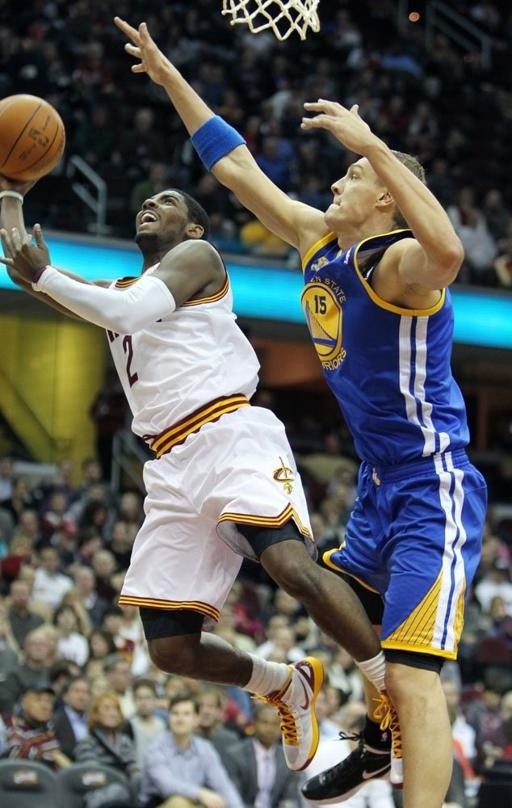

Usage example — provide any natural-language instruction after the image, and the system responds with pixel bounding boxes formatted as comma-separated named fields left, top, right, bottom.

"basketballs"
left=0, top=92, right=65, bottom=180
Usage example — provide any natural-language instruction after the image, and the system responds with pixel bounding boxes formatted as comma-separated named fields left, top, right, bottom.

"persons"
left=114, top=13, right=492, bottom=808
left=0, top=363, right=512, bottom=808
left=1, top=172, right=414, bottom=789
left=0, top=0, right=512, bottom=289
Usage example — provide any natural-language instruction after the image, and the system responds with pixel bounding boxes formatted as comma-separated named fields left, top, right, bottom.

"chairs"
left=0, top=483, right=511, bottom=807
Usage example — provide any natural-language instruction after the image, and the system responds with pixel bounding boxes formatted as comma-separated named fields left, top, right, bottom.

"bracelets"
left=1, top=190, right=26, bottom=204
left=186, top=114, right=249, bottom=170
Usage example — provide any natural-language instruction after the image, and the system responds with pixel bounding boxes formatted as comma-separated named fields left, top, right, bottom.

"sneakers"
left=250, top=656, right=404, bottom=805
